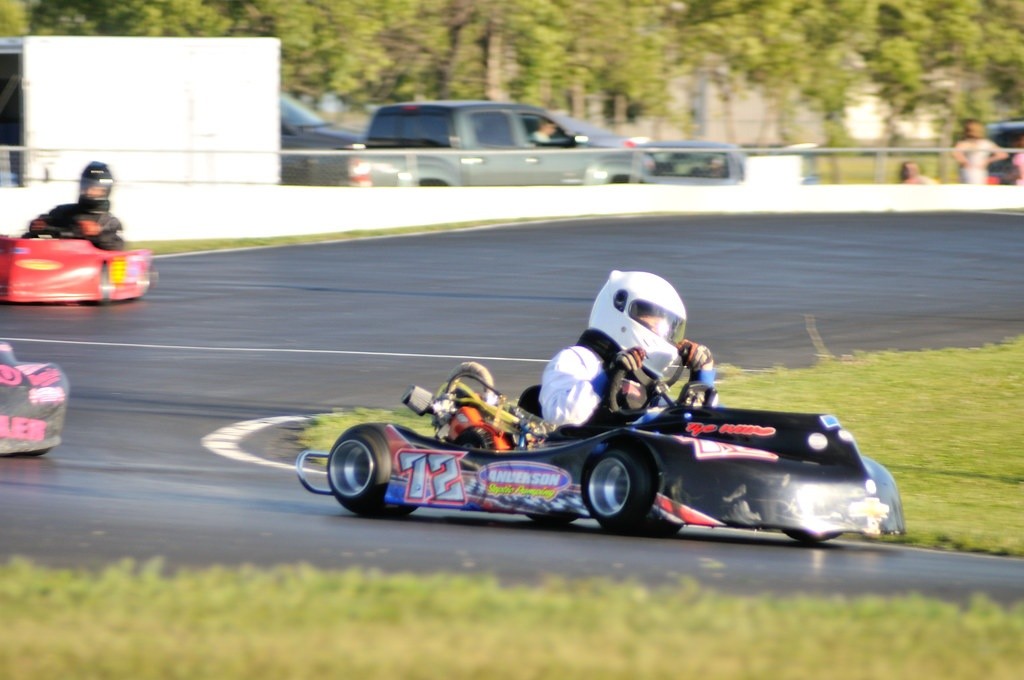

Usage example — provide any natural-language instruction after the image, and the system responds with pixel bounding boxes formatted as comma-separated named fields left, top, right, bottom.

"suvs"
left=350, top=98, right=655, bottom=190
left=277, top=93, right=374, bottom=190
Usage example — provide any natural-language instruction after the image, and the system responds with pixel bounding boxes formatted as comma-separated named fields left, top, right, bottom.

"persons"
left=947, top=117, right=1007, bottom=182
left=23, top=159, right=126, bottom=254
left=539, top=267, right=717, bottom=438
left=898, top=162, right=937, bottom=184
left=1010, top=132, right=1024, bottom=185
left=523, top=117, right=557, bottom=142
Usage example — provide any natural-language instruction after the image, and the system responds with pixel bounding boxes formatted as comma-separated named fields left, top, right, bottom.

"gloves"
left=73, top=219, right=104, bottom=236
left=676, top=337, right=716, bottom=373
left=27, top=219, right=49, bottom=234
left=606, top=346, right=647, bottom=378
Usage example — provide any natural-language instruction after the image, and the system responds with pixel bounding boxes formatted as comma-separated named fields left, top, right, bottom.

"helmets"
left=80, top=161, right=114, bottom=203
left=589, top=271, right=691, bottom=383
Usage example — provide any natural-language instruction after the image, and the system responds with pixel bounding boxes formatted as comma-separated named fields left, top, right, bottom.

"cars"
left=636, top=141, right=746, bottom=189
left=983, top=116, right=1024, bottom=187
